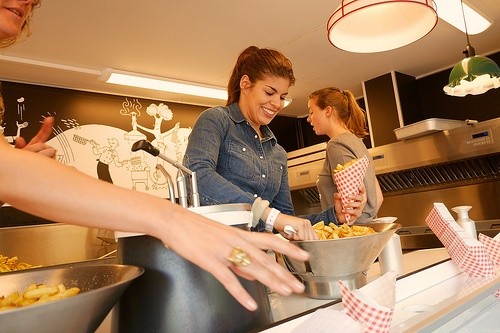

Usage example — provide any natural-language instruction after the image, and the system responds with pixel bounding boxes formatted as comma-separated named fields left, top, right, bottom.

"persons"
left=0, top=0, right=309, bottom=311
left=181, top=45, right=366, bottom=243
left=306, top=87, right=384, bottom=221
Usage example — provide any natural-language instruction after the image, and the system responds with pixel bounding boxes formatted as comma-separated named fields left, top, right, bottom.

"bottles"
left=450, top=206, right=478, bottom=241
left=373, top=215, right=404, bottom=278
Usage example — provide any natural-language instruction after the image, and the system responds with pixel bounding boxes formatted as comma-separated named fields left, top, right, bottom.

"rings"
left=227, top=249, right=251, bottom=268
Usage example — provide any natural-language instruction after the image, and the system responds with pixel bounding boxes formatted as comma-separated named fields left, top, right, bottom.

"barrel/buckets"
left=110, top=202, right=277, bottom=333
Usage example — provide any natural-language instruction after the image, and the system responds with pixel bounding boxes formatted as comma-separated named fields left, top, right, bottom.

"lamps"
left=98, top=67, right=292, bottom=109
left=433, top=0, right=494, bottom=36
left=443, top=0, right=500, bottom=97
left=327, top=0, right=438, bottom=54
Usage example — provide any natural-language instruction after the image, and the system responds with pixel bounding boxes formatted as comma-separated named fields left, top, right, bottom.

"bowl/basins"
left=273, top=222, right=403, bottom=300
left=0, top=263, right=146, bottom=333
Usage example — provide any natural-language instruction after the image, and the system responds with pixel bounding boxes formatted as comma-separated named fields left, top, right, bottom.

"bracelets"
left=265, top=208, right=281, bottom=233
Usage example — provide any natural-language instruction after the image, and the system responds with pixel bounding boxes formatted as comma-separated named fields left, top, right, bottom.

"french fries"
left=313, top=220, right=378, bottom=239
left=0, top=284, right=79, bottom=314
left=333, top=157, right=359, bottom=173
left=0, top=255, right=42, bottom=273
